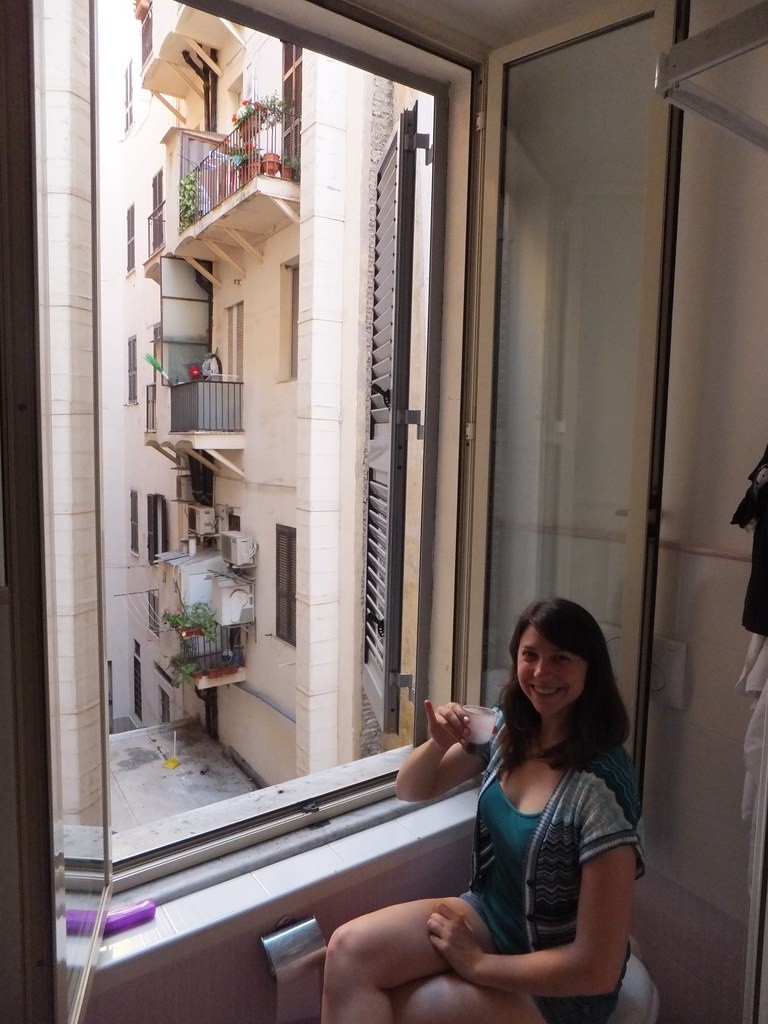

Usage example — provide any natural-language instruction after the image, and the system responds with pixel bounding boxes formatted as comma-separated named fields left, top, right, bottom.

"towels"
left=730, top=441, right=767, bottom=824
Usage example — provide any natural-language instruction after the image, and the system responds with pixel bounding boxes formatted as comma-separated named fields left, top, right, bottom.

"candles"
left=233, top=155, right=248, bottom=184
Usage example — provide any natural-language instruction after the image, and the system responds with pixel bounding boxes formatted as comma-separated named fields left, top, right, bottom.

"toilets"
left=600, top=935, right=661, bottom=1022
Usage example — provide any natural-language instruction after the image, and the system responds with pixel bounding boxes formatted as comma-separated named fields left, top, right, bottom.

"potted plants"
left=281, top=158, right=301, bottom=178
left=162, top=603, right=238, bottom=688
left=134, top=0, right=150, bottom=20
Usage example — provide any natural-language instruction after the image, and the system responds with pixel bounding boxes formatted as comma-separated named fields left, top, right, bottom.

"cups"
left=460, top=703, right=498, bottom=746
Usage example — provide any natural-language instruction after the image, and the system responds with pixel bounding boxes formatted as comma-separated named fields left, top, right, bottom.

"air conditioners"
left=176, top=476, right=193, bottom=501
left=187, top=505, right=215, bottom=534
left=222, top=530, right=254, bottom=565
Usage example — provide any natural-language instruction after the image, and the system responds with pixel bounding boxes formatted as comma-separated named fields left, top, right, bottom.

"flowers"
left=232, top=99, right=267, bottom=125
left=243, top=143, right=263, bottom=161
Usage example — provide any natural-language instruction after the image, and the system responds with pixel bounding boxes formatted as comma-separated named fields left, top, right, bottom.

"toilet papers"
left=272, top=968, right=322, bottom=1021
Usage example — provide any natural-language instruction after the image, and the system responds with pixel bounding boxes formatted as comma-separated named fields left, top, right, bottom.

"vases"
left=262, top=153, right=281, bottom=177
left=246, top=160, right=261, bottom=177
left=240, top=114, right=262, bottom=142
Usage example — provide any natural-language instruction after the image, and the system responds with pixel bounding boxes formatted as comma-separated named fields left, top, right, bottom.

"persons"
left=321, top=599, right=646, bottom=1024
